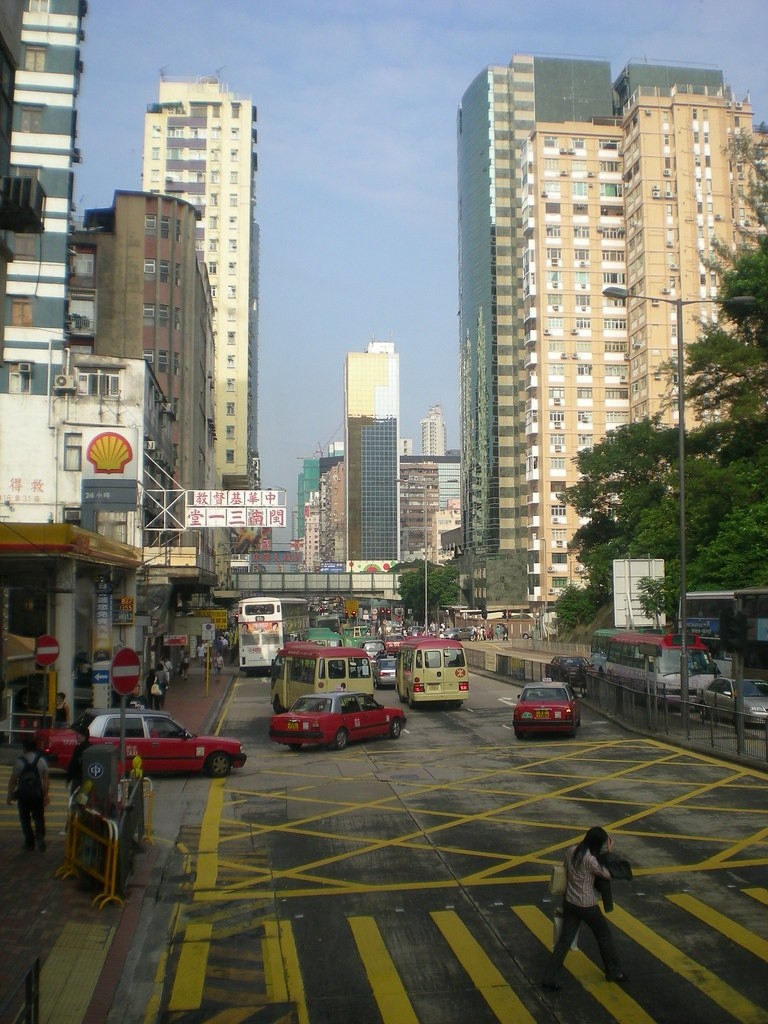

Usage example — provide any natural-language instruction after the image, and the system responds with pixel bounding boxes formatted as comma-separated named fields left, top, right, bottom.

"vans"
left=270, top=641, right=374, bottom=714
left=302, top=614, right=342, bottom=647
left=395, top=635, right=470, bottom=708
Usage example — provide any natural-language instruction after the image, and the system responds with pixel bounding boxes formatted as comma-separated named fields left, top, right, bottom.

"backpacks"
left=18, top=755, right=42, bottom=800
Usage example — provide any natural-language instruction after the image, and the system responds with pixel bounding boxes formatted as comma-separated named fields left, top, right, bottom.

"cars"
left=697, top=677, right=768, bottom=724
left=512, top=678, right=580, bottom=738
left=363, top=625, right=420, bottom=688
left=545, top=655, right=597, bottom=683
left=522, top=630, right=533, bottom=639
left=33, top=707, right=248, bottom=778
left=268, top=688, right=407, bottom=751
left=444, top=626, right=474, bottom=640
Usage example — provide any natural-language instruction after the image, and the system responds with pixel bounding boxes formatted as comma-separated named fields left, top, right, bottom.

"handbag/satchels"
left=548, top=845, right=578, bottom=895
left=552, top=916, right=578, bottom=951
left=151, top=677, right=162, bottom=696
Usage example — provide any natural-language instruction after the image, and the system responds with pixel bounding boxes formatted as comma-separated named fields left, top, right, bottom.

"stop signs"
left=110, top=648, right=141, bottom=694
left=33, top=635, right=60, bottom=665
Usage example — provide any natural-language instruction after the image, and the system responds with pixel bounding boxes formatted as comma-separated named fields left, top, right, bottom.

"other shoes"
left=58, top=831, right=66, bottom=836
left=23, top=844, right=34, bottom=851
left=40, top=843, right=46, bottom=852
left=606, top=973, right=629, bottom=981
left=543, top=982, right=567, bottom=991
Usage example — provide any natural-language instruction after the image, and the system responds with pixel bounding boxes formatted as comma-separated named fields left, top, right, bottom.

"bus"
left=590, top=628, right=722, bottom=710
left=237, top=597, right=310, bottom=668
left=678, top=588, right=768, bottom=680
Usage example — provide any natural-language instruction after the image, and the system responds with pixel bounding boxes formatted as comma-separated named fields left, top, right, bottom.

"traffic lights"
left=352, top=610, right=356, bottom=619
left=386, top=607, right=391, bottom=621
left=380, top=607, right=385, bottom=620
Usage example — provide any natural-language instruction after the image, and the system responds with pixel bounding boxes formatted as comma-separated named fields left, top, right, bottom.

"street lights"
left=602, top=287, right=759, bottom=742
left=393, top=478, right=458, bottom=636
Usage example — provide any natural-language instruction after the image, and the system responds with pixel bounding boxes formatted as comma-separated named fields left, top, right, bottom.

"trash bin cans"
left=80, top=744, right=121, bottom=815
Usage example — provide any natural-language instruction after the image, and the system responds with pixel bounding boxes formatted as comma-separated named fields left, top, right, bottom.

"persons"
left=58, top=727, right=93, bottom=837
left=127, top=685, right=149, bottom=710
left=430, top=620, right=445, bottom=631
left=55, top=692, right=71, bottom=728
left=176, top=630, right=231, bottom=683
left=147, top=656, right=172, bottom=710
left=241, top=623, right=249, bottom=634
left=470, top=623, right=508, bottom=642
left=543, top=826, right=626, bottom=991
left=270, top=622, right=278, bottom=633
left=7, top=738, right=50, bottom=852
